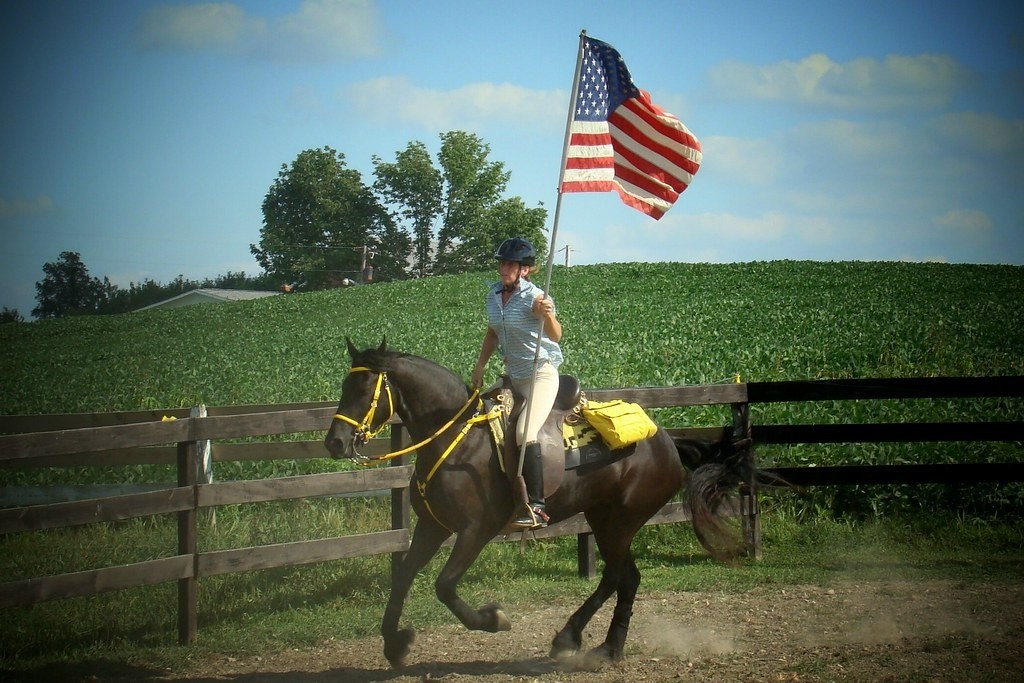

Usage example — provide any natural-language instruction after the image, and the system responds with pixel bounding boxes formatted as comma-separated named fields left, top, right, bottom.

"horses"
left=324, top=336, right=813, bottom=671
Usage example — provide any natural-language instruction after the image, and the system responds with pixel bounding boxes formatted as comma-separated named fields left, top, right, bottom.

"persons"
left=471, top=238, right=563, bottom=527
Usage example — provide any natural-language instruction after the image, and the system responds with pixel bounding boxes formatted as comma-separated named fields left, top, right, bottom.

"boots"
left=512, top=441, right=548, bottom=530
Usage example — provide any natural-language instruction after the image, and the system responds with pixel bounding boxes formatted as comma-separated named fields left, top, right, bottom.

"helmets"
left=495, top=236, right=535, bottom=267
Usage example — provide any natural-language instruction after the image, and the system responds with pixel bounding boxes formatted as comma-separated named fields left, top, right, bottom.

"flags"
left=559, top=36, right=702, bottom=220
